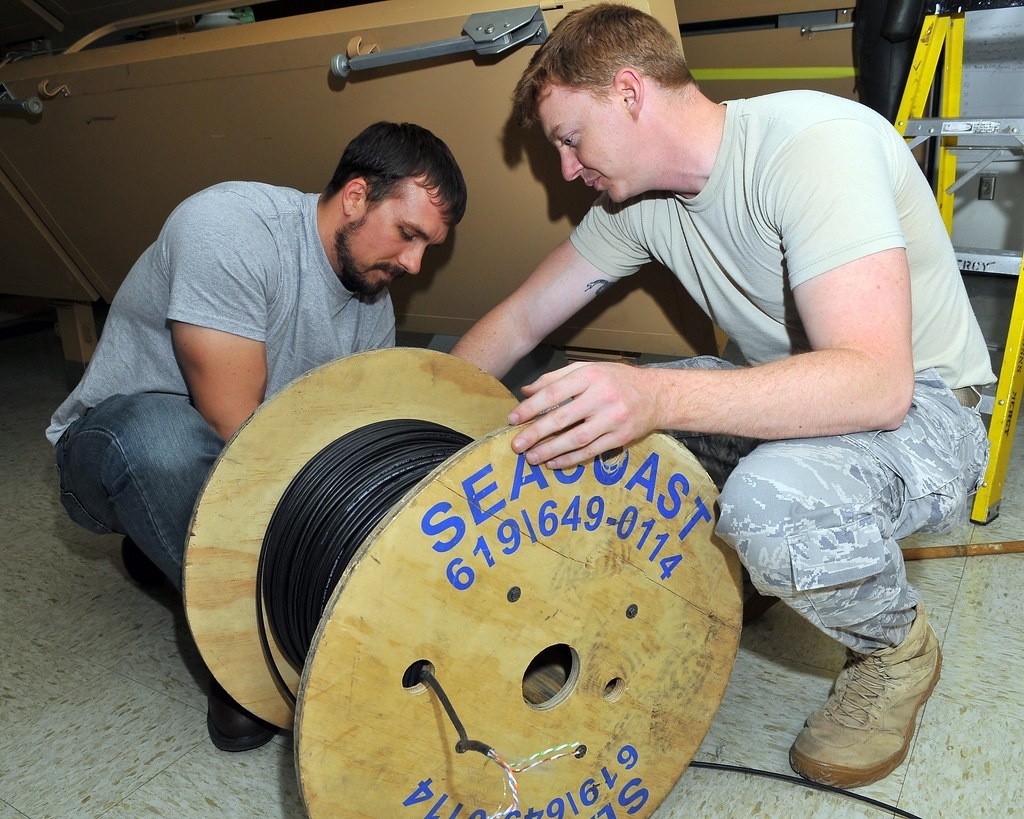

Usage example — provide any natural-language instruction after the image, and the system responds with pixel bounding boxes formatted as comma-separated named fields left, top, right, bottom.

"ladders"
left=891, top=1, right=1024, bottom=525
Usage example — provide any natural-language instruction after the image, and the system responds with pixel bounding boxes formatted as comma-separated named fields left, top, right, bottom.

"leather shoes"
left=201, top=674, right=276, bottom=752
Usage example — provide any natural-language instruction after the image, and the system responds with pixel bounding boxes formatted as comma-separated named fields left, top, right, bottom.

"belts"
left=954, top=384, right=985, bottom=411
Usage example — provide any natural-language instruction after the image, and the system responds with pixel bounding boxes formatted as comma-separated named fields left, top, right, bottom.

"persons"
left=45, top=117, right=470, bottom=753
left=444, top=3, right=1002, bottom=791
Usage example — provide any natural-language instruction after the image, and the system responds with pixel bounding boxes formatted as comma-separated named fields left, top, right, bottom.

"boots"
left=788, top=601, right=947, bottom=784
